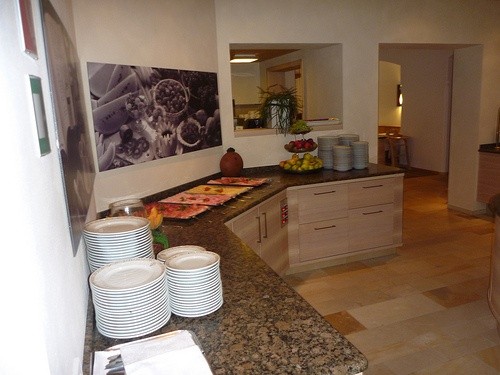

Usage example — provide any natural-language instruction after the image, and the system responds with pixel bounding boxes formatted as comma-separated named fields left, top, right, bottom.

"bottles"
left=220, top=148, right=243, bottom=176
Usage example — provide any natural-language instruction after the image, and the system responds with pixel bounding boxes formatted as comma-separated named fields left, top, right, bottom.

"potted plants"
left=261, top=87, right=302, bottom=128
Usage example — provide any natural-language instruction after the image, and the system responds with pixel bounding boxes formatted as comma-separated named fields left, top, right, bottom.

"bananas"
left=147, top=208, right=163, bottom=230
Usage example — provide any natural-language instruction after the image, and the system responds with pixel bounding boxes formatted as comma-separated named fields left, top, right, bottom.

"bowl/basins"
left=154, top=80, right=188, bottom=117
left=181, top=71, right=218, bottom=98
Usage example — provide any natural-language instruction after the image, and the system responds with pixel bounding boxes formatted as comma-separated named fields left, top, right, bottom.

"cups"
left=234, top=117, right=263, bottom=129
left=177, top=118, right=202, bottom=146
left=110, top=199, right=148, bottom=219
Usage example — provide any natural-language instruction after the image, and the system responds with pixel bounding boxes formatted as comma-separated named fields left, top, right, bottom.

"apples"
left=279, top=153, right=323, bottom=171
left=284, top=138, right=318, bottom=151
left=239, top=111, right=261, bottom=121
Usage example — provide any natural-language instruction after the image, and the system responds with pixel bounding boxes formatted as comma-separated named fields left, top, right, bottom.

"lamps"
left=397, top=84, right=405, bottom=106
left=230, top=53, right=260, bottom=65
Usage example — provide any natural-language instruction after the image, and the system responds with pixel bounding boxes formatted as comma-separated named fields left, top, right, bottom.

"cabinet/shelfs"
left=227, top=191, right=292, bottom=275
left=298, top=175, right=406, bottom=262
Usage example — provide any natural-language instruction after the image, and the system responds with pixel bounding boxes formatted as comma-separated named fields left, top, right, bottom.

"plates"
left=159, top=193, right=238, bottom=206
left=83, top=216, right=155, bottom=272
left=318, top=134, right=369, bottom=171
left=89, top=257, right=171, bottom=339
left=207, top=176, right=269, bottom=186
left=145, top=201, right=211, bottom=219
left=157, top=245, right=223, bottom=318
left=183, top=184, right=253, bottom=195
